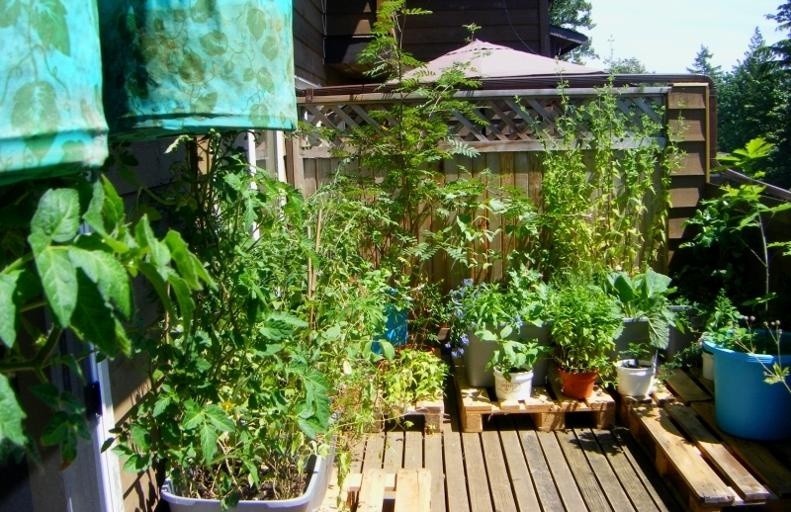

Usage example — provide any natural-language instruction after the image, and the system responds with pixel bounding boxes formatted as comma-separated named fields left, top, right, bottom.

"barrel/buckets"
left=371, top=287, right=408, bottom=355
left=613, top=359, right=656, bottom=397
left=701, top=351, right=714, bottom=381
left=493, top=365, right=533, bottom=401
left=701, top=328, right=791, bottom=440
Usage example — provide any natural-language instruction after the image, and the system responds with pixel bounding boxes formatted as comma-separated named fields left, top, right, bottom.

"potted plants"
left=447, top=264, right=785, bottom=441
left=144, top=150, right=410, bottom=509
